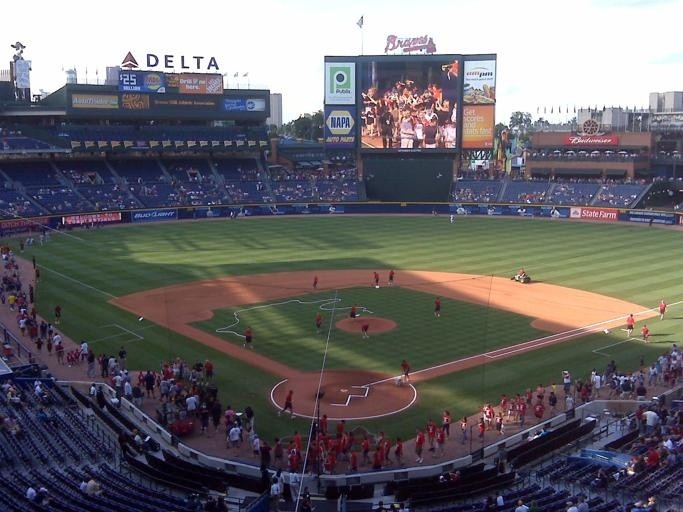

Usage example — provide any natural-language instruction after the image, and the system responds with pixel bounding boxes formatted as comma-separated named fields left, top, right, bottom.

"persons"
left=312, top=274, right=318, bottom=288
left=627, top=313, right=635, bottom=336
left=432, top=294, right=442, bottom=318
left=245, top=325, right=253, bottom=351
left=659, top=300, right=667, bottom=321
left=641, top=324, right=649, bottom=341
left=518, top=267, right=527, bottom=276
left=373, top=267, right=397, bottom=289
left=1, top=218, right=64, bottom=342
left=316, top=304, right=371, bottom=344
left=362, top=59, right=494, bottom=149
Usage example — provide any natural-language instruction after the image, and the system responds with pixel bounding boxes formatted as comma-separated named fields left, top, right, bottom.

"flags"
left=356, top=16, right=363, bottom=28
left=536, top=103, right=652, bottom=115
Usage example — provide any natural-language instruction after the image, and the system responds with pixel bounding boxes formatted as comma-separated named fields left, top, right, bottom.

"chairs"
left=1, top=378, right=228, bottom=510
left=1, top=125, right=358, bottom=222
left=382, top=404, right=682, bottom=511
left=658, top=154, right=679, bottom=159
left=450, top=177, right=646, bottom=209
left=324, top=482, right=375, bottom=499
left=532, top=152, right=640, bottom=160
